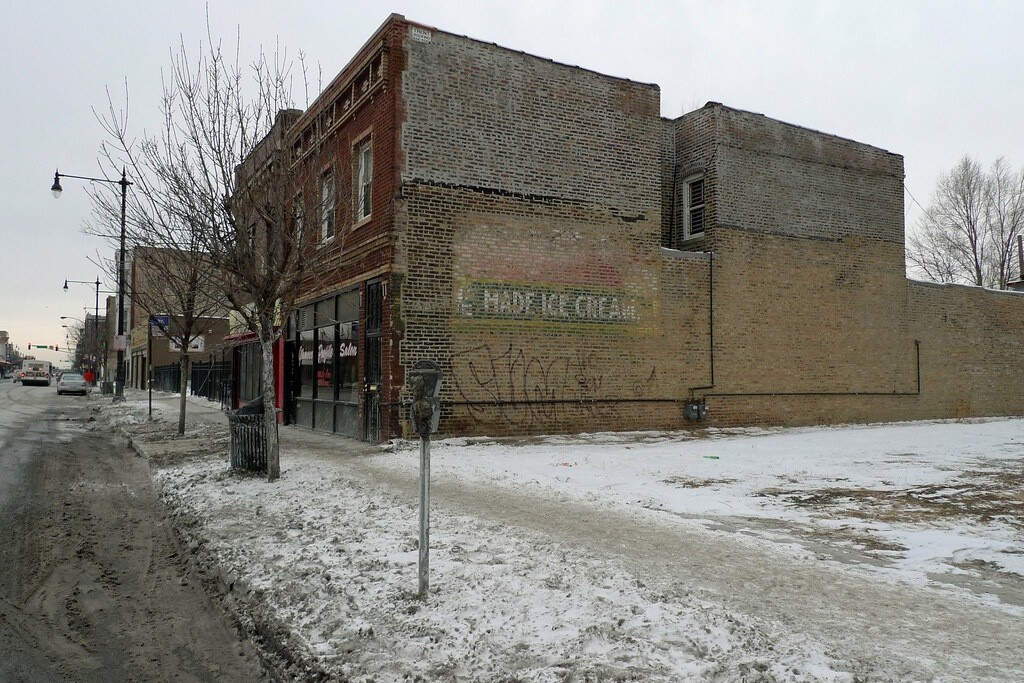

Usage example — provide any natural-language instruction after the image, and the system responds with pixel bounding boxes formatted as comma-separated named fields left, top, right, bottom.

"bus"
left=21, top=360, right=55, bottom=386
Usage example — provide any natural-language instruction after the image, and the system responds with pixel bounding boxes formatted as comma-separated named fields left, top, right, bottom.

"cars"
left=56, top=372, right=87, bottom=396
left=4, top=369, right=22, bottom=383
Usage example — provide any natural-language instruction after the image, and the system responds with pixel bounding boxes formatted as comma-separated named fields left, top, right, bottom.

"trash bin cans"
left=223, top=409, right=283, bottom=476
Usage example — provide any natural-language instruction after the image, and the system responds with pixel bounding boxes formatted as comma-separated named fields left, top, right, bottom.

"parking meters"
left=406, top=357, right=445, bottom=600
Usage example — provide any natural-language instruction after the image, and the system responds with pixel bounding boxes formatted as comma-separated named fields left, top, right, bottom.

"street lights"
left=63, top=274, right=102, bottom=388
left=51, top=166, right=134, bottom=402
left=60, top=316, right=87, bottom=335
